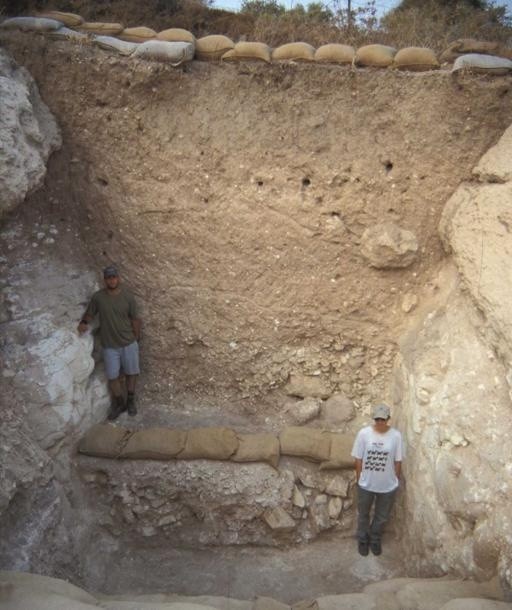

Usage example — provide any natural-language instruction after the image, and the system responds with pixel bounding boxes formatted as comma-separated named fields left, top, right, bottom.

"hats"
left=373, top=404, right=389, bottom=419
left=103, top=267, right=118, bottom=279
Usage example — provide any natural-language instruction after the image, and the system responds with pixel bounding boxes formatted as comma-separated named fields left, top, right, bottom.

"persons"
left=350, top=403, right=403, bottom=556
left=78, top=266, right=141, bottom=421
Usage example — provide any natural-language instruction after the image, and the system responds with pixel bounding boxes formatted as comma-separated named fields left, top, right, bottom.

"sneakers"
left=126, top=399, right=137, bottom=415
left=371, top=543, right=381, bottom=554
left=110, top=405, right=125, bottom=418
left=360, top=543, right=369, bottom=555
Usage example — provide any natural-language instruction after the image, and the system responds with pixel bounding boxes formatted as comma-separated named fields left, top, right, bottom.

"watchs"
left=80, top=320, right=87, bottom=324
